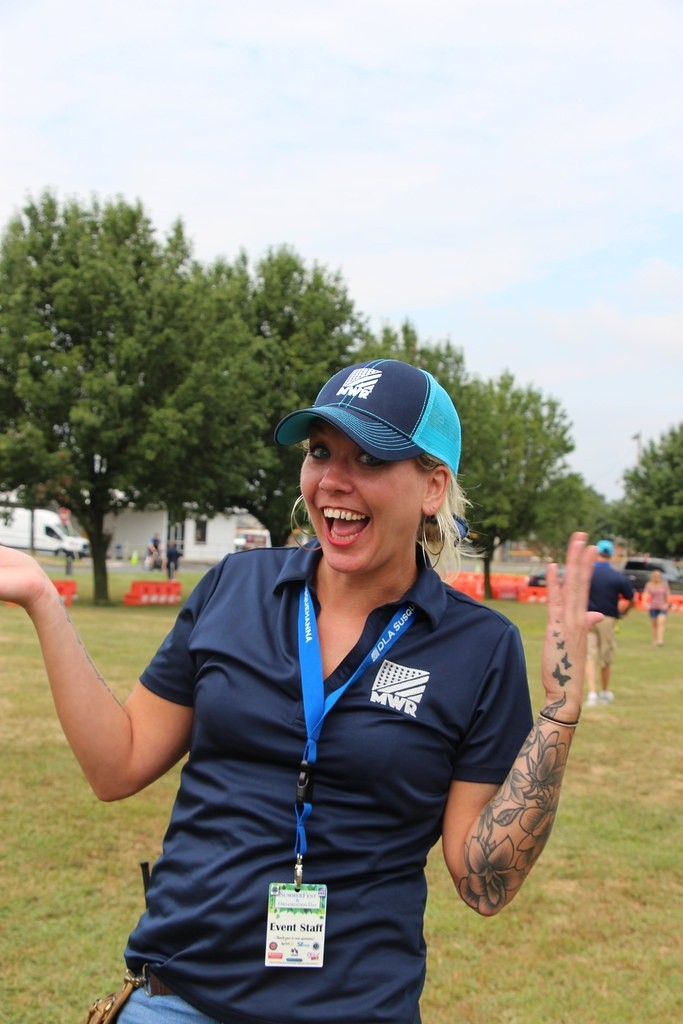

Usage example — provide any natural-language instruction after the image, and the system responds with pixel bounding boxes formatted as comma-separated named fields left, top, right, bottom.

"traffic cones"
left=130, top=549, right=136, bottom=563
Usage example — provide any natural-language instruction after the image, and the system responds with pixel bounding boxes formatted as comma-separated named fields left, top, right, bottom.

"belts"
left=142, top=964, right=174, bottom=996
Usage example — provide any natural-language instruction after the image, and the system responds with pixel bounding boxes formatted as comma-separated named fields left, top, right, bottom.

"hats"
left=596, top=540, right=614, bottom=556
left=272, top=359, right=462, bottom=476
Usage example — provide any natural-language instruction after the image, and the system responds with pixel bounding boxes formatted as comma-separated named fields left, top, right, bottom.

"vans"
left=623, top=558, right=681, bottom=589
left=0, top=504, right=89, bottom=561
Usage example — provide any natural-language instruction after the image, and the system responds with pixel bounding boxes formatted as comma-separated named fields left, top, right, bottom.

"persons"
left=146, top=533, right=163, bottom=569
left=585, top=540, right=633, bottom=708
left=166, top=545, right=183, bottom=579
left=0, top=361, right=602, bottom=1024
left=641, top=570, right=670, bottom=644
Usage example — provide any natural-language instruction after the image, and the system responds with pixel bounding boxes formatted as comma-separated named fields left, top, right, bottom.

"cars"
left=532, top=568, right=565, bottom=586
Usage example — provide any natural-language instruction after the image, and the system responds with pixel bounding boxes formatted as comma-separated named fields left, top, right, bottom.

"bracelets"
left=539, top=712, right=580, bottom=727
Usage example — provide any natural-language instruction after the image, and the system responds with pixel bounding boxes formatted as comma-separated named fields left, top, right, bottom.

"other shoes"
left=654, top=640, right=662, bottom=646
left=600, top=692, right=613, bottom=705
left=587, top=694, right=597, bottom=706
